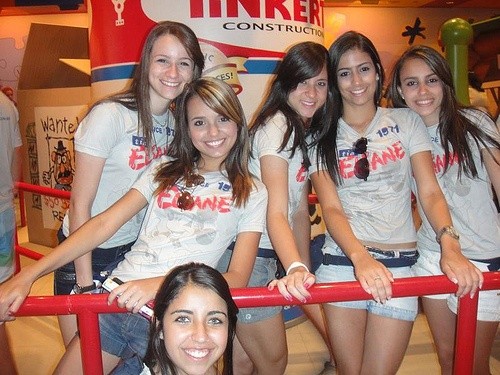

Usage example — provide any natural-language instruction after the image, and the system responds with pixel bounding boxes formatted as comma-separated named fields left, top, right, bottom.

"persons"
left=109, top=261, right=240, bottom=375
left=217, top=39, right=337, bottom=375
left=0, top=74, right=269, bottom=375
left=0, top=87, right=23, bottom=284
left=304, top=31, right=483, bottom=375
left=388, top=45, right=500, bottom=375
left=50, top=20, right=207, bottom=354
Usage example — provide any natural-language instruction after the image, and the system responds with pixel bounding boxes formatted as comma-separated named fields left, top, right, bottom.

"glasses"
left=352, top=137, right=369, bottom=181
left=177, top=174, right=205, bottom=212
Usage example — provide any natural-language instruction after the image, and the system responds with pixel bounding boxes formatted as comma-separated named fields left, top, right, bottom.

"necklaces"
left=144, top=110, right=169, bottom=156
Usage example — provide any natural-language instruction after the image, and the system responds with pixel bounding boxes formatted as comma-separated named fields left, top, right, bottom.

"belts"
left=470, top=257, right=500, bottom=271
left=227, top=242, right=277, bottom=258
left=322, top=252, right=419, bottom=267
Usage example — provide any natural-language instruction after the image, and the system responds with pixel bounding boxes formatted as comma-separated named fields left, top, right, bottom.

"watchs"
left=72, top=281, right=97, bottom=295
left=435, top=225, right=460, bottom=244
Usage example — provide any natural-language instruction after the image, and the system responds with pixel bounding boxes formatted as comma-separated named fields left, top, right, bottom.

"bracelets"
left=286, top=261, right=310, bottom=276
